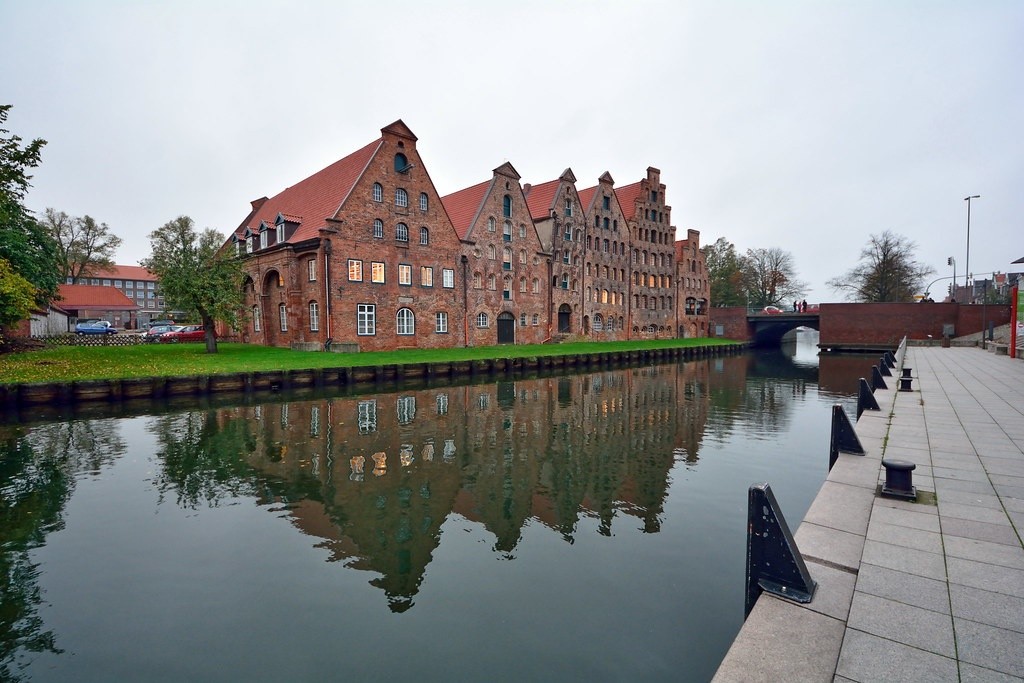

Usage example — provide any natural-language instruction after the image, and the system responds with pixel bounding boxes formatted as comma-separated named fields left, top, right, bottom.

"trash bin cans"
left=942, top=337, right=950, bottom=348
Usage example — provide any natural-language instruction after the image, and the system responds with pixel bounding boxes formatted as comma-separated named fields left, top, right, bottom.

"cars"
left=147, top=325, right=218, bottom=342
left=142, top=319, right=175, bottom=328
left=75, top=322, right=118, bottom=336
left=761, top=306, right=784, bottom=315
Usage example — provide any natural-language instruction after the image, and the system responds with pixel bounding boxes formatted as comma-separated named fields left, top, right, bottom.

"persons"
left=920, top=297, right=957, bottom=303
left=794, top=299, right=807, bottom=313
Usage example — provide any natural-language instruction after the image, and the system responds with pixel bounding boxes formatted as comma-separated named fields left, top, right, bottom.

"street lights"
left=947, top=256, right=957, bottom=290
left=964, top=194, right=981, bottom=289
